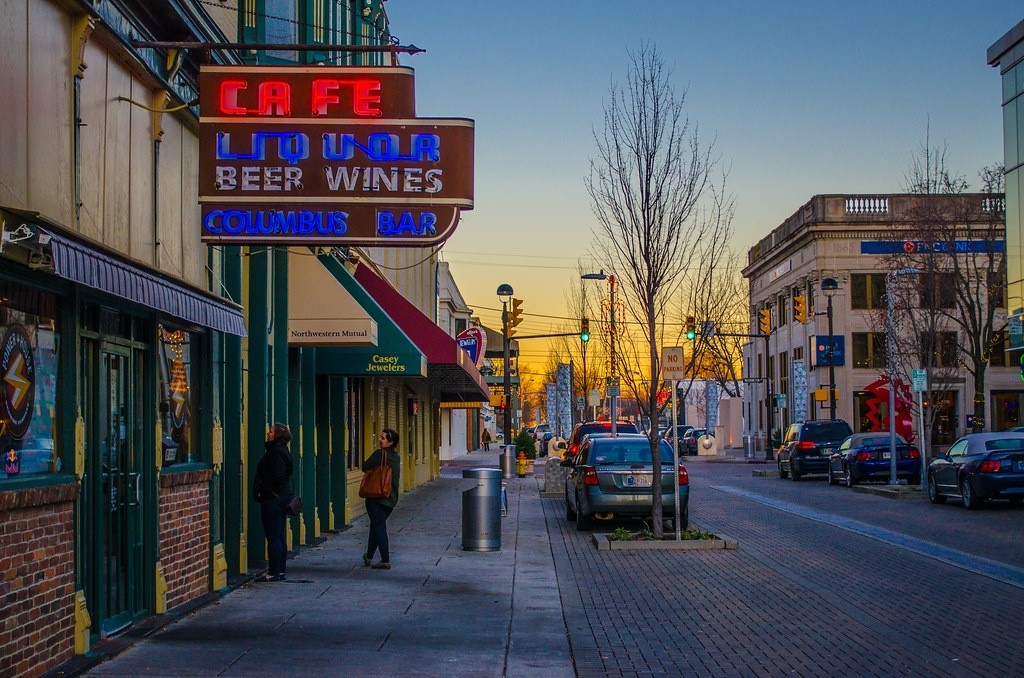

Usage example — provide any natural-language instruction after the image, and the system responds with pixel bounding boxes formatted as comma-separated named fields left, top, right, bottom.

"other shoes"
left=373, top=560, right=391, bottom=569
left=363, top=552, right=372, bottom=566
left=254, top=572, right=287, bottom=582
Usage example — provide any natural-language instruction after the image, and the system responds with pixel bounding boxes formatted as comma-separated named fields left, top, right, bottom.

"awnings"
left=287, top=245, right=491, bottom=403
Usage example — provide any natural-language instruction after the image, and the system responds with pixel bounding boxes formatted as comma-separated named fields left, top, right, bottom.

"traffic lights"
left=758, top=308, right=771, bottom=335
left=686, top=315, right=695, bottom=340
left=507, top=311, right=517, bottom=337
left=581, top=316, right=590, bottom=342
left=513, top=298, right=524, bottom=327
left=794, top=295, right=806, bottom=323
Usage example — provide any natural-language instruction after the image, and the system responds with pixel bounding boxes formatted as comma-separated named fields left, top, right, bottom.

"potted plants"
left=772, top=428, right=781, bottom=459
left=515, top=429, right=535, bottom=473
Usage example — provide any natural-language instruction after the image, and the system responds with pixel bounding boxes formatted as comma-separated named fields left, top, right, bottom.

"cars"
left=525, top=423, right=552, bottom=458
left=926, top=432, right=1024, bottom=510
left=828, top=432, right=923, bottom=488
left=560, top=436, right=690, bottom=532
left=562, top=433, right=647, bottom=459
left=639, top=425, right=714, bottom=457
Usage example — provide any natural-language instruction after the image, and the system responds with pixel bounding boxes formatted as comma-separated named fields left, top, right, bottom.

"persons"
left=362, top=429, right=400, bottom=567
left=255, top=423, right=294, bottom=582
left=482, top=428, right=491, bottom=451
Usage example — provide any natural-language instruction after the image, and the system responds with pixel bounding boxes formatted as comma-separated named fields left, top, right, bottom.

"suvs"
left=776, top=419, right=853, bottom=481
left=558, top=420, right=640, bottom=451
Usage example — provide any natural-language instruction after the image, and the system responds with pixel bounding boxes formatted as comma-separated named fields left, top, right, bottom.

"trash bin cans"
left=498, top=445, right=517, bottom=479
left=461, top=468, right=502, bottom=552
left=743, top=435, right=755, bottom=458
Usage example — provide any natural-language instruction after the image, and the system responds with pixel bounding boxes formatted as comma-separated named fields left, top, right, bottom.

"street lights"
left=884, top=267, right=928, bottom=484
left=579, top=268, right=617, bottom=435
left=496, top=283, right=513, bottom=444
left=821, top=276, right=837, bottom=420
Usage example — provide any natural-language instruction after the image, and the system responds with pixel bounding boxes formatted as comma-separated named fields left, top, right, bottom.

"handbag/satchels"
left=281, top=495, right=302, bottom=516
left=359, top=449, right=391, bottom=499
